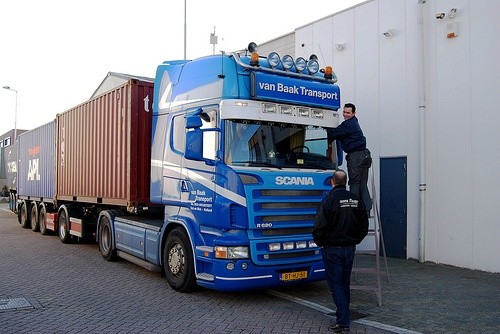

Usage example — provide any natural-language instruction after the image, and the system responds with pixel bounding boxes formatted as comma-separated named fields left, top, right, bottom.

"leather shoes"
left=328, top=324, right=350, bottom=334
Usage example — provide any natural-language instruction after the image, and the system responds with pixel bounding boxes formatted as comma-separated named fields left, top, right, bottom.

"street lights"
left=2, top=86, right=17, bottom=139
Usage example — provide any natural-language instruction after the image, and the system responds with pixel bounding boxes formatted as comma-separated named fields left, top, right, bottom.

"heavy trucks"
left=17, top=42, right=343, bottom=295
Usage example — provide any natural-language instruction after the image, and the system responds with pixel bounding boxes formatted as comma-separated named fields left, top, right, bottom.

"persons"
left=312, top=170, right=373, bottom=334
left=327, top=102, right=373, bottom=217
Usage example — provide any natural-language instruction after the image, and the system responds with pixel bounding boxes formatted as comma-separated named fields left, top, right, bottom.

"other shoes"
left=366, top=210, right=370, bottom=218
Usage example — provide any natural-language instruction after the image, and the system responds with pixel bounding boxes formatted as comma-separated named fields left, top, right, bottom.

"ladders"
left=350, top=162, right=392, bottom=307
